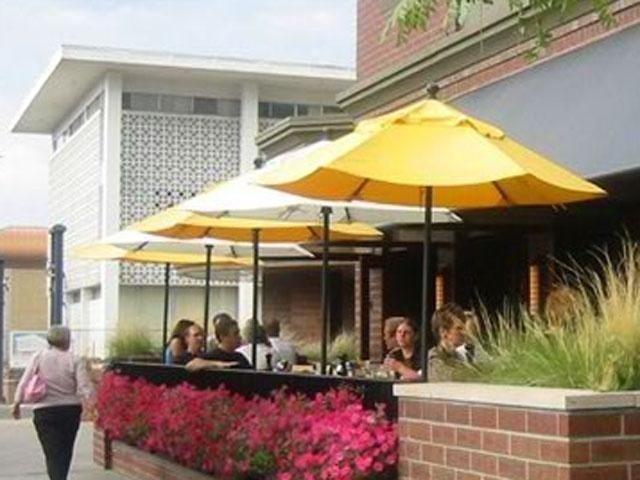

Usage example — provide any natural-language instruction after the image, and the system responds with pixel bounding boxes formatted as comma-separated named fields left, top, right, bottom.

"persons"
left=11, top=325, right=99, bottom=480
left=163, top=303, right=481, bottom=381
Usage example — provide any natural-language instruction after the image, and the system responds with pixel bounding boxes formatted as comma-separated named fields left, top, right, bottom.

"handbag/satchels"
left=22, top=374, right=47, bottom=403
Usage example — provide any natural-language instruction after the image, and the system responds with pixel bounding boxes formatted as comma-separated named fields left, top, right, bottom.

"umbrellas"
left=179, top=137, right=464, bottom=373
left=255, top=81, right=609, bottom=381
